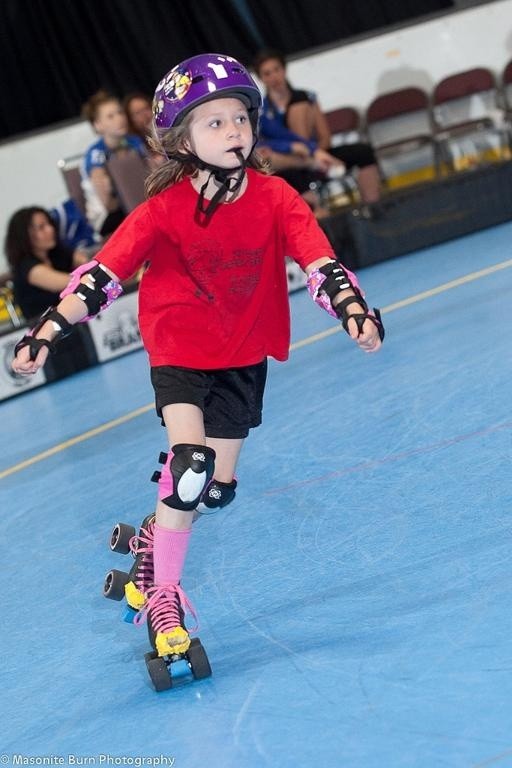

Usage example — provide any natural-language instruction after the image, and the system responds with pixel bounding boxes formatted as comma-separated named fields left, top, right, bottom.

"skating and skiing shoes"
left=103, top=511, right=156, bottom=611
left=143, top=580, right=212, bottom=691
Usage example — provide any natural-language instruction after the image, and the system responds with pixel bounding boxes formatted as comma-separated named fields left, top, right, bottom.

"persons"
left=9, top=50, right=387, bottom=665
left=252, top=111, right=346, bottom=174
left=122, top=91, right=175, bottom=168
left=84, top=87, right=156, bottom=239
left=4, top=204, right=106, bottom=382
left=254, top=48, right=382, bottom=219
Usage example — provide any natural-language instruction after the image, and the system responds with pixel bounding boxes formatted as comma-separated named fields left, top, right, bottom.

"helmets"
left=150, top=52, right=265, bottom=164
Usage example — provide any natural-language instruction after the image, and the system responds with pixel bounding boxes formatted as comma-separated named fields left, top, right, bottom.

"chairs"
left=319, top=59, right=512, bottom=206
